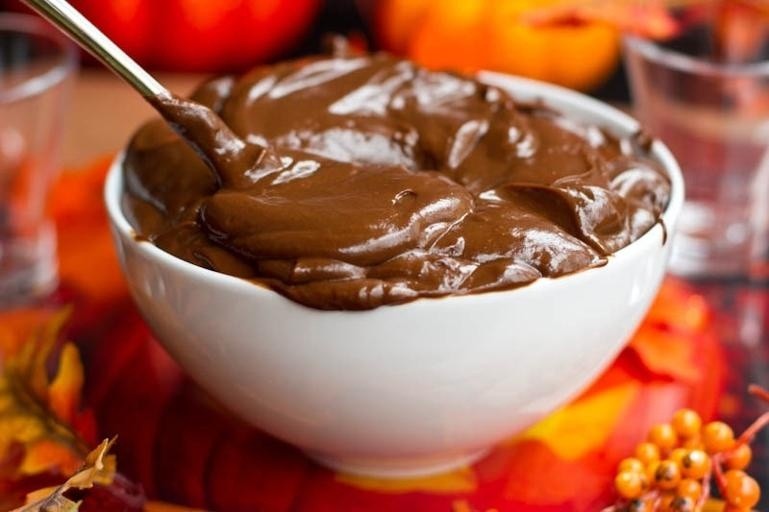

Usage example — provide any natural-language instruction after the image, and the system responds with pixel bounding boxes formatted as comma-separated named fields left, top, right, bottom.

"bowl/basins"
left=102, top=68, right=687, bottom=481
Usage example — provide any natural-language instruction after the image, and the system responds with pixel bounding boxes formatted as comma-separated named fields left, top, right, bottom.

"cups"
left=623, top=35, right=768, bottom=283
left=1, top=14, right=78, bottom=305
